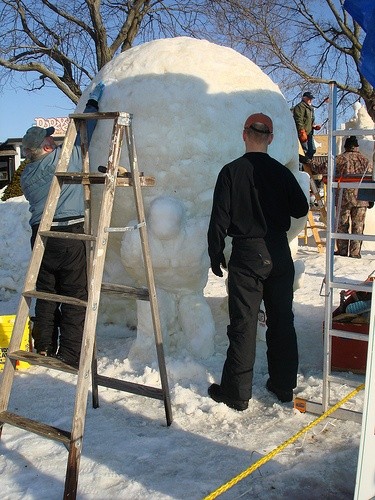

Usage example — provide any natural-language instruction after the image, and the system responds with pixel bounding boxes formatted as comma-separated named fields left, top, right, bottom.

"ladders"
left=0, top=111, right=174, bottom=500
left=297, top=138, right=327, bottom=254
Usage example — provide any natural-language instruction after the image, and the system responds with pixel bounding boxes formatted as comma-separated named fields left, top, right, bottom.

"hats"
left=22, top=126, right=55, bottom=157
left=244, top=113, right=273, bottom=134
left=303, top=92, right=315, bottom=99
left=344, top=136, right=359, bottom=148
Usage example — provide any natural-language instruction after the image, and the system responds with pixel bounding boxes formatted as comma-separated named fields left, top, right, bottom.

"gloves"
left=210, top=253, right=227, bottom=277
left=299, top=130, right=307, bottom=143
left=367, top=201, right=374, bottom=209
left=313, top=124, right=321, bottom=130
left=89, top=81, right=106, bottom=101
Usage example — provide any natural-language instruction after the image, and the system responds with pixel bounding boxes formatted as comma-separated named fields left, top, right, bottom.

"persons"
left=208, top=112, right=309, bottom=411
left=19, top=80, right=105, bottom=371
left=299, top=136, right=374, bottom=258
left=293, top=92, right=322, bottom=160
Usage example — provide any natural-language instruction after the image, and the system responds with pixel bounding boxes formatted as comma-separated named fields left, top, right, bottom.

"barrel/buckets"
left=0, top=314, right=33, bottom=371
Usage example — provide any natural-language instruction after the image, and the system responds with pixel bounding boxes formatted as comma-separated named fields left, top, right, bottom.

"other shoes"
left=334, top=250, right=340, bottom=255
left=208, top=383, right=249, bottom=411
left=266, top=378, right=294, bottom=403
left=350, top=254, right=361, bottom=258
